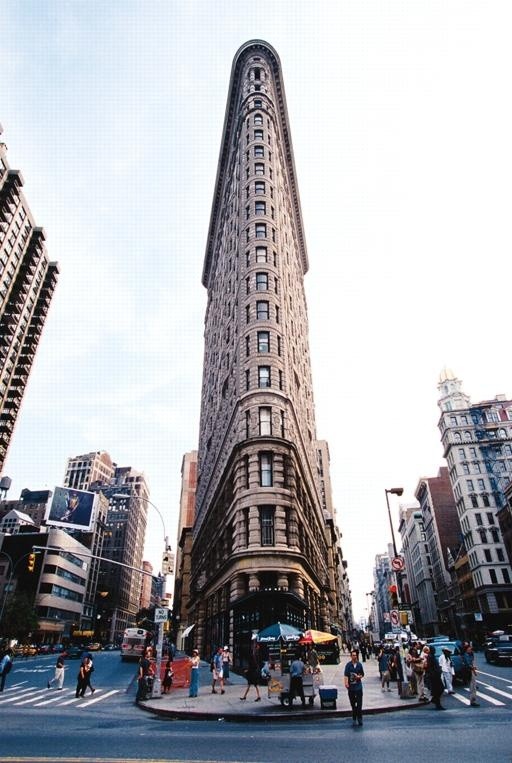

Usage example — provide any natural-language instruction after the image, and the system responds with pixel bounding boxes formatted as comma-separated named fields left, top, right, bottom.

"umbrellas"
left=256, top=621, right=303, bottom=662
left=298, top=629, right=337, bottom=664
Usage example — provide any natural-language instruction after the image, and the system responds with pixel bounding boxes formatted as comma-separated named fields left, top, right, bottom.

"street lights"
left=0, top=550, right=42, bottom=619
left=384, top=486, right=416, bottom=632
left=112, top=492, right=168, bottom=550
left=366, top=591, right=372, bottom=628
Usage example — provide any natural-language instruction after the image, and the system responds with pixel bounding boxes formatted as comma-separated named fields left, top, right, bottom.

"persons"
left=189, top=649, right=200, bottom=697
left=211, top=645, right=232, bottom=694
left=308, top=647, right=319, bottom=675
left=342, top=638, right=480, bottom=727
left=75, top=654, right=95, bottom=698
left=240, top=656, right=262, bottom=702
left=48, top=652, right=68, bottom=690
left=0, top=650, right=11, bottom=691
left=289, top=655, right=305, bottom=708
left=136, top=647, right=175, bottom=701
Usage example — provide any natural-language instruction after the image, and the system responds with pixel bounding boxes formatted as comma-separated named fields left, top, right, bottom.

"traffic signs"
left=162, top=551, right=175, bottom=575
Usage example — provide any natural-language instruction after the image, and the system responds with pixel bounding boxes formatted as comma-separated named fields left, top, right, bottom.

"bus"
left=119, top=626, right=148, bottom=658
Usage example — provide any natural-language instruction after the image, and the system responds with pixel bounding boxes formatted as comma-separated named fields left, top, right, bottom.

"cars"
left=13, top=641, right=119, bottom=659
left=481, top=630, right=512, bottom=666
left=374, top=630, right=472, bottom=686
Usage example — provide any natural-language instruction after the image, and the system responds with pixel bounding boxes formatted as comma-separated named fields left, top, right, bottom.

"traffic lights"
left=390, top=585, right=400, bottom=607
left=27, top=552, right=36, bottom=574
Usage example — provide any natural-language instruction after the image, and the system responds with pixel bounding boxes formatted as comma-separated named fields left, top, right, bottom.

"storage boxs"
left=319, top=688, right=337, bottom=699
left=321, top=699, right=336, bottom=710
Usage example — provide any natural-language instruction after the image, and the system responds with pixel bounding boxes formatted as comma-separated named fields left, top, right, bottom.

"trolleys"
left=268, top=672, right=317, bottom=706
